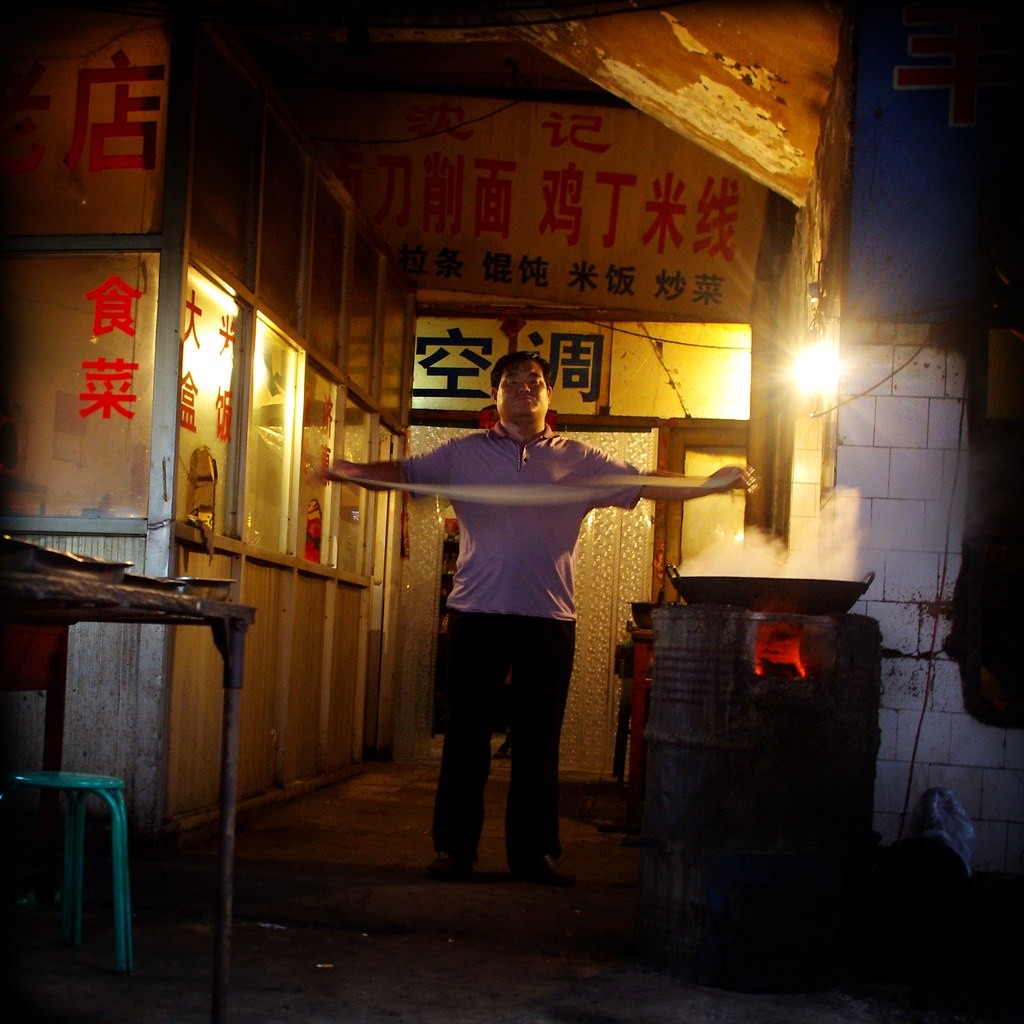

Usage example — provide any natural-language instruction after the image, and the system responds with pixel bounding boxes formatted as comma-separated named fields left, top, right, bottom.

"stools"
left=0, top=772, right=133, bottom=976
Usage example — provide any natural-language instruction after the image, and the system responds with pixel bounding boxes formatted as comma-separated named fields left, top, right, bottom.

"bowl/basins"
left=178, top=576, right=237, bottom=602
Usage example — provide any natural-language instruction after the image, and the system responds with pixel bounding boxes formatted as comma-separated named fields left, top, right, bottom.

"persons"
left=333, top=350, right=757, bottom=885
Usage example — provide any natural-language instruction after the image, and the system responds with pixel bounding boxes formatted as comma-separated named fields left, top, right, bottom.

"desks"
left=0, top=568, right=260, bottom=1023
left=624, top=631, right=653, bottom=828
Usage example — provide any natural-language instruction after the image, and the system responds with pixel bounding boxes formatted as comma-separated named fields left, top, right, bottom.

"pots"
left=665, top=562, right=876, bottom=615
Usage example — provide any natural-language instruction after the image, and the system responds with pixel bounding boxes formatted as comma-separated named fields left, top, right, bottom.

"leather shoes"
left=426, top=851, right=475, bottom=881
left=508, top=854, right=574, bottom=888
